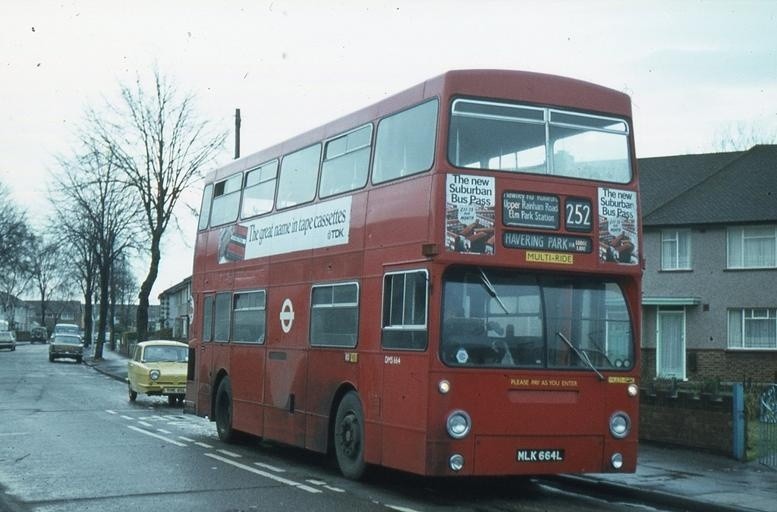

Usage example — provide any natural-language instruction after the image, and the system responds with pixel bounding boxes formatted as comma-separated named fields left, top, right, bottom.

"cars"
left=0, top=322, right=110, bottom=364
left=124, top=338, right=189, bottom=405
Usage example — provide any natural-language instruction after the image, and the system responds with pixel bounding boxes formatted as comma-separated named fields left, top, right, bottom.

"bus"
left=182, top=67, right=647, bottom=486
